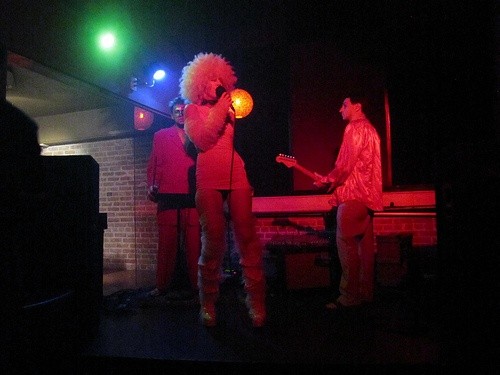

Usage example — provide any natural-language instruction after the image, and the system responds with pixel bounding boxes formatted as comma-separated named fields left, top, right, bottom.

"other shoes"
left=358, top=292, right=374, bottom=303
left=146, top=287, right=168, bottom=296
left=250, top=308, right=266, bottom=327
left=326, top=295, right=362, bottom=309
left=201, top=303, right=216, bottom=325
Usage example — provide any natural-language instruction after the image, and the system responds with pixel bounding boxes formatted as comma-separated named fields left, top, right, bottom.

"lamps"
left=228, top=89, right=254, bottom=118
left=141, top=63, right=165, bottom=87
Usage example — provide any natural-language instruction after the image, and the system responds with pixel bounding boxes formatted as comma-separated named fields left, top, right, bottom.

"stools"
left=269, top=240, right=336, bottom=296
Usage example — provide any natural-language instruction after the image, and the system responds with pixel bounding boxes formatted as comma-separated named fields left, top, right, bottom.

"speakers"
left=283, top=250, right=332, bottom=291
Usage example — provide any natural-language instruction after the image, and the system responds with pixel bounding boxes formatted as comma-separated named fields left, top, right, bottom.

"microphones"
left=215, top=85, right=236, bottom=113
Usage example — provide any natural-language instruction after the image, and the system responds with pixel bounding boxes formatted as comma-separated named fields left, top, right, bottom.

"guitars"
left=275, top=153, right=338, bottom=207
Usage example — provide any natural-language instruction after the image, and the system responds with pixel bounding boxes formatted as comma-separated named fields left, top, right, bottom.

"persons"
left=146, top=96, right=201, bottom=292
left=178, top=52, right=266, bottom=326
left=313, top=93, right=382, bottom=310
